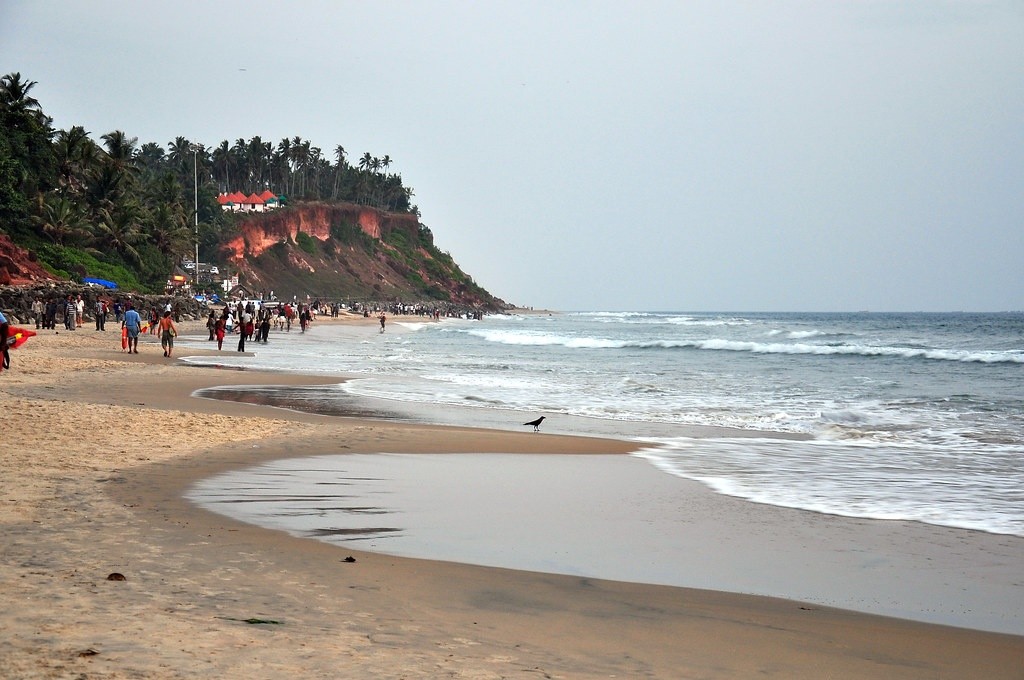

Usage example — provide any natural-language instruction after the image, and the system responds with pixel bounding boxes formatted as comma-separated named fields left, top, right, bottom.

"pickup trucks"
left=209, top=267, right=220, bottom=274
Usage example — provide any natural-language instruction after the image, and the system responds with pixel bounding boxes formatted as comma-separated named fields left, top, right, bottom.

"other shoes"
left=42, top=328, right=45, bottom=329
left=36, top=328, right=39, bottom=329
left=51, top=328, right=55, bottom=330
left=101, top=329, right=105, bottom=331
left=76, top=326, right=81, bottom=327
left=96, top=329, right=99, bottom=330
left=70, top=329, right=75, bottom=330
left=47, top=328, right=50, bottom=329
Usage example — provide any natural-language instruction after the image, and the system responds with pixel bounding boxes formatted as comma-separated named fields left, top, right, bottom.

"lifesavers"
left=122, top=328, right=127, bottom=349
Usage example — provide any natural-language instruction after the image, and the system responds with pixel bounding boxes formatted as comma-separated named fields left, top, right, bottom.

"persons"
left=121, top=306, right=142, bottom=354
left=93, top=296, right=133, bottom=331
left=206, top=297, right=344, bottom=352
left=0, top=312, right=10, bottom=369
left=32, top=295, right=84, bottom=330
left=358, top=300, right=481, bottom=331
left=148, top=299, right=178, bottom=358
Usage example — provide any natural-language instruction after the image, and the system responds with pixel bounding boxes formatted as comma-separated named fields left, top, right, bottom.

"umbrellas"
left=226, top=201, right=235, bottom=210
left=264, top=195, right=286, bottom=212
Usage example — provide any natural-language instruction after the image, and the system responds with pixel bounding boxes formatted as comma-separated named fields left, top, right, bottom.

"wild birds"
left=523, top=416, right=546, bottom=431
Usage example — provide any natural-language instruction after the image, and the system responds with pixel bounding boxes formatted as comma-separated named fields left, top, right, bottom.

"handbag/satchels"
left=168, top=323, right=174, bottom=337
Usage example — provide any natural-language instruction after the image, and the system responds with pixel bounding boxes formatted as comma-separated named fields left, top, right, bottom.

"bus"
left=186, top=263, right=206, bottom=272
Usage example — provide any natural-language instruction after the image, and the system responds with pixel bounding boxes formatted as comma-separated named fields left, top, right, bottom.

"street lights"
left=189, top=148, right=201, bottom=283
